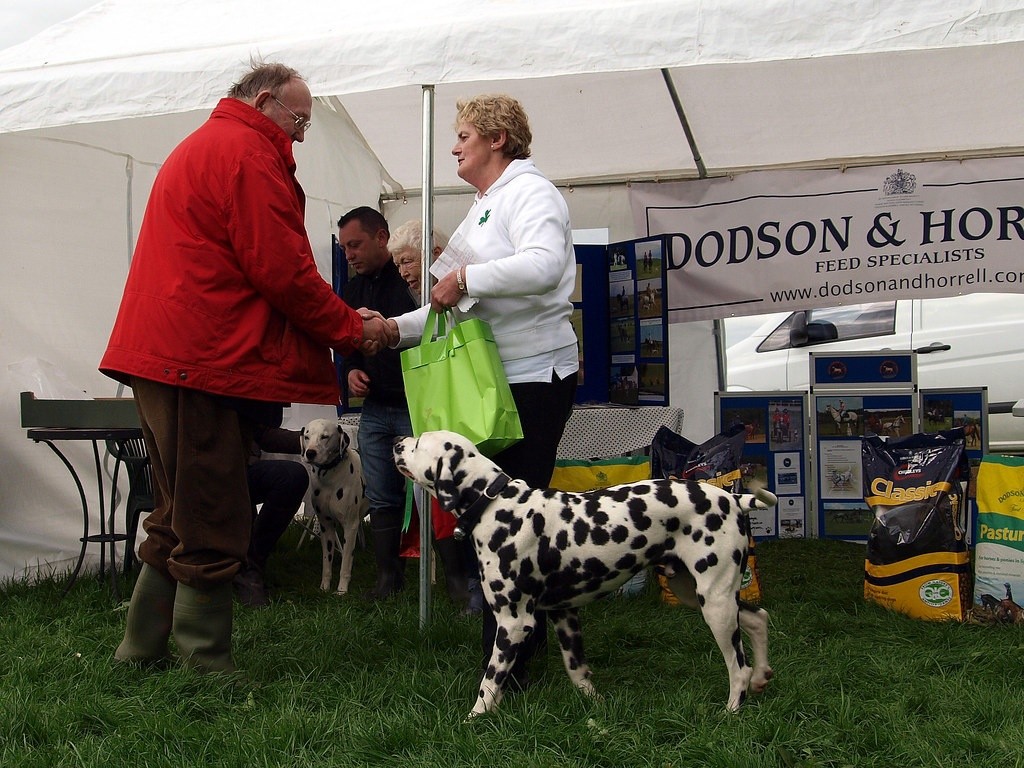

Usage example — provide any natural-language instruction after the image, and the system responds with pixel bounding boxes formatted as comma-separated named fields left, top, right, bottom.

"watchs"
left=457, top=266, right=468, bottom=294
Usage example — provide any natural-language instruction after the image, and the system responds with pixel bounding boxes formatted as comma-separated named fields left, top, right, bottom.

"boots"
left=114, top=561, right=236, bottom=674
left=368, top=522, right=404, bottom=599
left=433, top=534, right=482, bottom=619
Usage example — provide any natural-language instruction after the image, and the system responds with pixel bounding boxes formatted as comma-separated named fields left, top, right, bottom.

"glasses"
left=271, top=94, right=311, bottom=132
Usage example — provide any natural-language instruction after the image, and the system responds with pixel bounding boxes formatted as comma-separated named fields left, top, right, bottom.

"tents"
left=0, top=3, right=1022, bottom=590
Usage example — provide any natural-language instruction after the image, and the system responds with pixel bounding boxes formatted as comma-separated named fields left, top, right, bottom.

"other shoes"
left=236, top=579, right=266, bottom=606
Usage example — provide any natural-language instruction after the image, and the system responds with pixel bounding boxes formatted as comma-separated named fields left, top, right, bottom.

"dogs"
left=392, top=429, right=779, bottom=722
left=300, top=418, right=364, bottom=596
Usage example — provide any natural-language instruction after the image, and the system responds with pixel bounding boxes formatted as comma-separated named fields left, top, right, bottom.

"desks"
left=20, top=389, right=145, bottom=586
left=302, top=405, right=685, bottom=594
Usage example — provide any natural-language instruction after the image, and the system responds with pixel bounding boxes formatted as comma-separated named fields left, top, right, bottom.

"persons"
left=729, top=415, right=756, bottom=439
left=773, top=408, right=790, bottom=436
left=648, top=332, right=652, bottom=347
left=614, top=247, right=625, bottom=266
left=247, top=427, right=306, bottom=609
left=611, top=380, right=637, bottom=398
left=647, top=283, right=653, bottom=304
left=98, top=63, right=393, bottom=676
left=359, top=95, right=580, bottom=695
left=621, top=286, right=626, bottom=298
left=338, top=207, right=475, bottom=607
left=387, top=218, right=451, bottom=307
left=839, top=399, right=846, bottom=423
left=617, top=321, right=633, bottom=344
left=927, top=406, right=945, bottom=425
left=962, top=414, right=968, bottom=428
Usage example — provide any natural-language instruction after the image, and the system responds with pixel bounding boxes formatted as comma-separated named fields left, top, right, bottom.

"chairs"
left=106, top=439, right=162, bottom=578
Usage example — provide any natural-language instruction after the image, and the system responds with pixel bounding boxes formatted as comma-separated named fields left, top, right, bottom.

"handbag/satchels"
left=400, top=304, right=525, bottom=459
